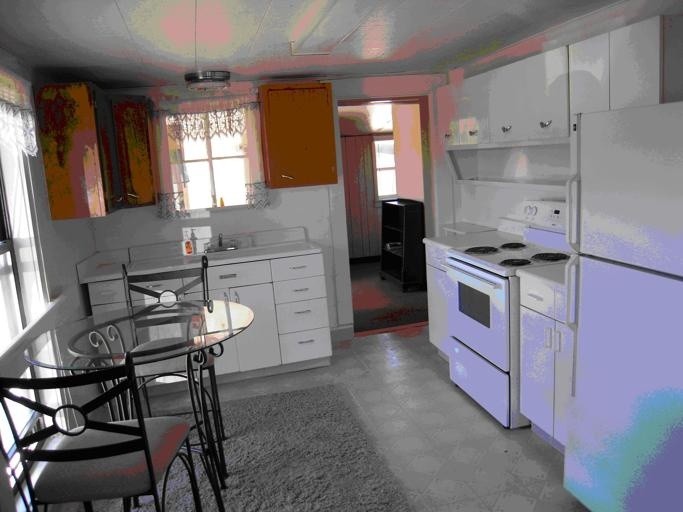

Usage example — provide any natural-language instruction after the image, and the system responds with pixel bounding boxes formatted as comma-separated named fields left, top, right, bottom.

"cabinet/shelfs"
left=380, top=197, right=424, bottom=292
left=433, top=73, right=488, bottom=150
left=516, top=260, right=579, bottom=457
left=261, top=78, right=338, bottom=191
left=490, top=45, right=568, bottom=149
left=76, top=226, right=331, bottom=403
left=423, top=222, right=523, bottom=361
left=33, top=78, right=159, bottom=221
left=568, top=16, right=681, bottom=114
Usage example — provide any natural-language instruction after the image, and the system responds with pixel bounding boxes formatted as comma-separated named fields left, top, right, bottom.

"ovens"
left=446, top=259, right=510, bottom=373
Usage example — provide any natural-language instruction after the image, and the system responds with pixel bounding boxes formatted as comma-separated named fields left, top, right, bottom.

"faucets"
left=219, top=233, right=222, bottom=248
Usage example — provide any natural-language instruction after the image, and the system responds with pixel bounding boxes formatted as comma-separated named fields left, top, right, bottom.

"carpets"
left=29, top=385, right=411, bottom=512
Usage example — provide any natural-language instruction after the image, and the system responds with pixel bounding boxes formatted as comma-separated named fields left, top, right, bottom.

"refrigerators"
left=564, top=101, right=682, bottom=511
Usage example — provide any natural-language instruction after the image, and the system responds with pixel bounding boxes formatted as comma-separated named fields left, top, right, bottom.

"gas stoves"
left=452, top=241, right=570, bottom=269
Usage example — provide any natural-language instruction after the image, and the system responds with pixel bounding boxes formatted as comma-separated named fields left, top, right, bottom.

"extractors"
left=444, top=143, right=570, bottom=184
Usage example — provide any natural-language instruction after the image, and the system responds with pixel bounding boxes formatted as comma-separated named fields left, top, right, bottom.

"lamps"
left=184, top=70, right=231, bottom=93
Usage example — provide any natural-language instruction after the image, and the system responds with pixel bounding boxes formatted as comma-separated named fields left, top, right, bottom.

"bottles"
left=219, top=197, right=224, bottom=207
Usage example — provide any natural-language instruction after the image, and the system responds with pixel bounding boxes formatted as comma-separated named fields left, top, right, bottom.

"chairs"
left=119, top=257, right=225, bottom=444
left=1, top=352, right=202, bottom=512
